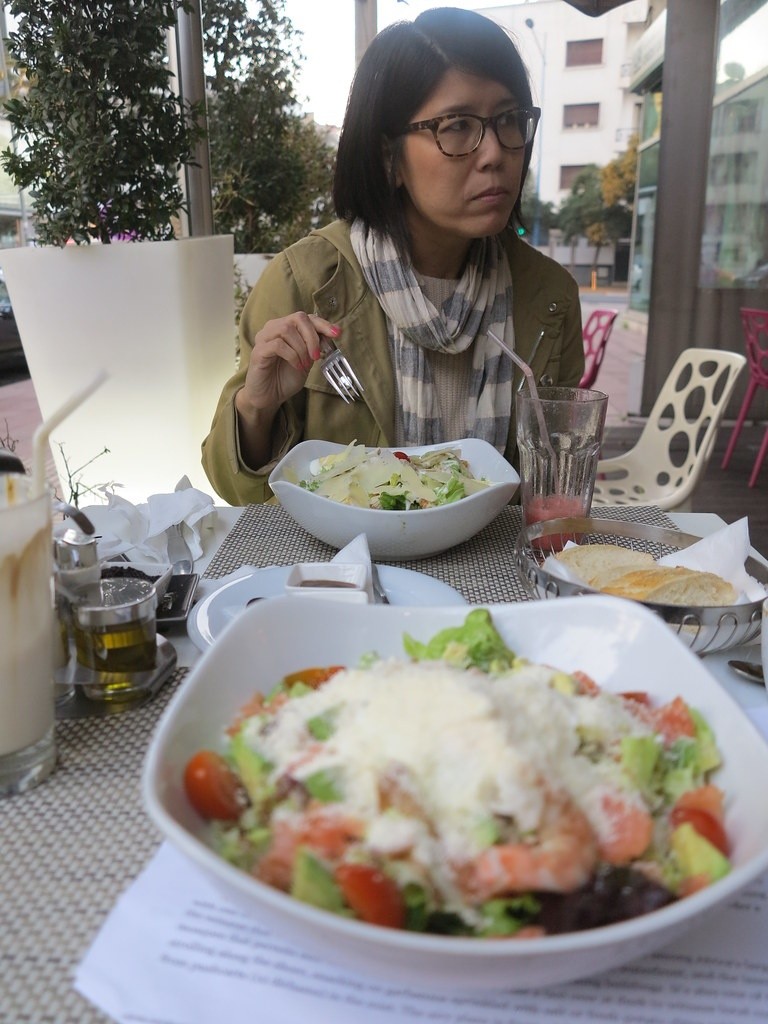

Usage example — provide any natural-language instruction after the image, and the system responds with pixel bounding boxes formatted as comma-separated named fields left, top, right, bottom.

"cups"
left=73, top=578, right=157, bottom=702
left=0, top=473, right=55, bottom=794
left=760, top=598, right=768, bottom=692
left=516, top=387, right=609, bottom=551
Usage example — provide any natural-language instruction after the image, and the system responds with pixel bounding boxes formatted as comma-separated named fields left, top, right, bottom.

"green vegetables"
left=298, top=469, right=465, bottom=511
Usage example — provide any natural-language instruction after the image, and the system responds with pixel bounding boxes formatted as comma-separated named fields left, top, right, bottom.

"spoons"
left=726, top=657, right=764, bottom=684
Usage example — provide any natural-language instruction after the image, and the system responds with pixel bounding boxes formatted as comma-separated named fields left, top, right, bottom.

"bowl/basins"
left=137, top=595, right=768, bottom=990
left=101, top=554, right=173, bottom=597
left=285, top=563, right=367, bottom=591
left=268, top=438, right=520, bottom=561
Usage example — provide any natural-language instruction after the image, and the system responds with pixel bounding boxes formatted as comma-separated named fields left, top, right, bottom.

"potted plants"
left=0, top=0, right=310, bottom=511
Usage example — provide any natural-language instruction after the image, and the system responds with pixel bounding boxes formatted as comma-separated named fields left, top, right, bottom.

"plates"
left=155, top=574, right=200, bottom=621
left=187, top=566, right=467, bottom=654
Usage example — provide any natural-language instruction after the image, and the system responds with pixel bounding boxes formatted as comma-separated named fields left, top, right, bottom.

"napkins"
left=64, top=475, right=219, bottom=563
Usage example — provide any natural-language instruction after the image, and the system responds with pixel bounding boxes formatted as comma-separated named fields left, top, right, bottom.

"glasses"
left=405, top=105, right=542, bottom=157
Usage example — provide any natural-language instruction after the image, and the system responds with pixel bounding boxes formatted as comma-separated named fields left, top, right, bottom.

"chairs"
left=593, top=346, right=748, bottom=508
left=721, top=308, right=768, bottom=488
left=581, top=308, right=618, bottom=389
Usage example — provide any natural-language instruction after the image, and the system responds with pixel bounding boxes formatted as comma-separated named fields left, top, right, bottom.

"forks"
left=317, top=333, right=364, bottom=405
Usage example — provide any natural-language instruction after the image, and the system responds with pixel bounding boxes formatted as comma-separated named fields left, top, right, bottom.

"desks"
left=1, top=503, right=767, bottom=1024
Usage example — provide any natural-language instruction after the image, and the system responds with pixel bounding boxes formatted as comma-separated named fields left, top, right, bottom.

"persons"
left=201, top=8, right=585, bottom=507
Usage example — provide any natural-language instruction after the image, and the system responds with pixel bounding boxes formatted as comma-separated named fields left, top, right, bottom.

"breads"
left=540, top=545, right=737, bottom=634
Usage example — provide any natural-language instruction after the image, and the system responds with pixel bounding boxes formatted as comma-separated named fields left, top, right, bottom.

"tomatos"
left=185, top=666, right=732, bottom=934
